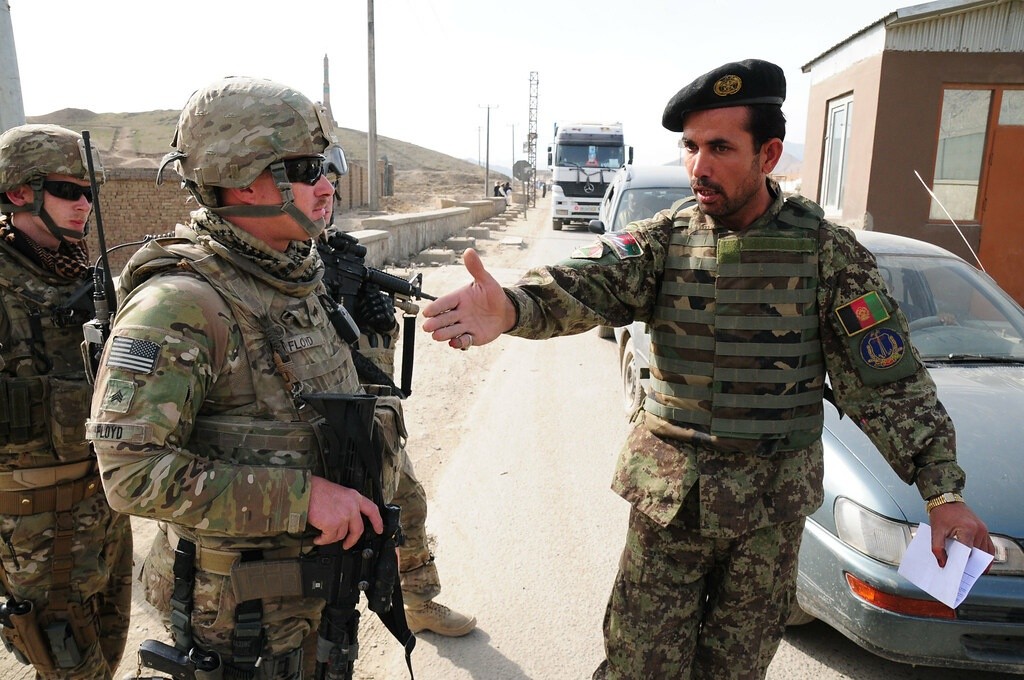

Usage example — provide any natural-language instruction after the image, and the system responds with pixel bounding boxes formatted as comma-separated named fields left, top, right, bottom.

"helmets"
left=0, top=124, right=105, bottom=193
left=170, top=74, right=338, bottom=188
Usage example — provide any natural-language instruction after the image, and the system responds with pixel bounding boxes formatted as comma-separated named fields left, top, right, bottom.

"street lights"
left=479, top=104, right=500, bottom=197
left=507, top=122, right=519, bottom=185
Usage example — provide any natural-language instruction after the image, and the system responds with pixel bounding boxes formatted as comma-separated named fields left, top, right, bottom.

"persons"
left=493, top=180, right=513, bottom=207
left=84, top=75, right=409, bottom=680
left=0, top=121, right=134, bottom=680
left=422, top=57, right=996, bottom=680
left=877, top=265, right=957, bottom=327
left=542, top=183, right=546, bottom=198
left=315, top=143, right=478, bottom=640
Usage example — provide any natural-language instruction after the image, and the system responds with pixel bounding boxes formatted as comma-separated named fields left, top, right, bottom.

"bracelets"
left=926, top=492, right=965, bottom=516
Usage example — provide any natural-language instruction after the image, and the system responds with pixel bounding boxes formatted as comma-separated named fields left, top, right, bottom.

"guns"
left=140, top=640, right=220, bottom=680
left=0, top=599, right=31, bottom=629
left=317, top=232, right=438, bottom=349
left=299, top=393, right=405, bottom=680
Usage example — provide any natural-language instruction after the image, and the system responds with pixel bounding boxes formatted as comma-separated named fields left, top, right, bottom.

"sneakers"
left=404, top=599, right=477, bottom=637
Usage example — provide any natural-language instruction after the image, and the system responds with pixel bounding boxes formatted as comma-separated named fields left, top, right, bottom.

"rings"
left=461, top=334, right=473, bottom=351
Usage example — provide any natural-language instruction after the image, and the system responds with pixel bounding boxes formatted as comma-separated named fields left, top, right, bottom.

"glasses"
left=322, top=145, right=348, bottom=175
left=44, top=181, right=93, bottom=203
left=266, top=157, right=326, bottom=186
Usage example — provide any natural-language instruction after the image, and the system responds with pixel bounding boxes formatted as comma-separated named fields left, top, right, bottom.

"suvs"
left=589, top=163, right=694, bottom=338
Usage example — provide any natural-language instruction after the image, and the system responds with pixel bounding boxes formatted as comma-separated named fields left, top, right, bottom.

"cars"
left=612, top=230, right=1023, bottom=675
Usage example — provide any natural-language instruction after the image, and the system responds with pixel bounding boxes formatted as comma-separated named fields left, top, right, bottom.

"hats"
left=662, top=59, right=787, bottom=133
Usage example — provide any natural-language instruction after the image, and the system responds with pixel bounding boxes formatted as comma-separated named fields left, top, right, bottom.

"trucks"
left=547, top=123, right=633, bottom=230
